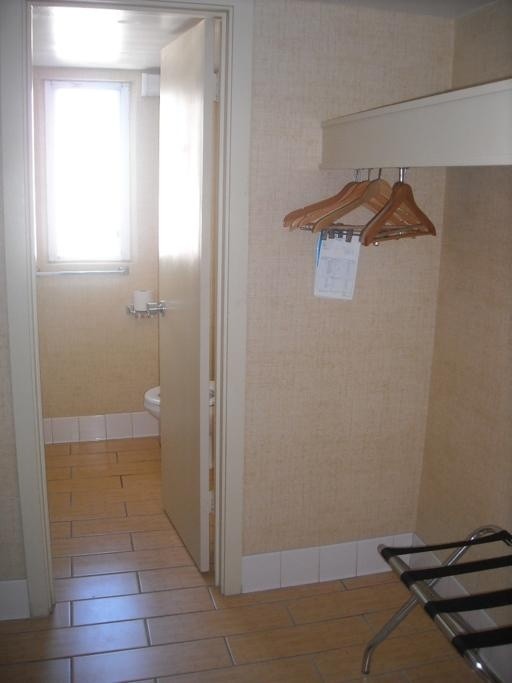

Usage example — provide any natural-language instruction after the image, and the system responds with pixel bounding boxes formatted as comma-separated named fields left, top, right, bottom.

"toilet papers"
left=133, top=290, right=154, bottom=311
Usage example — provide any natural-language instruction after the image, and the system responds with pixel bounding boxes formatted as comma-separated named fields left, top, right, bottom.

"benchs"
left=357, top=524, right=512, bottom=682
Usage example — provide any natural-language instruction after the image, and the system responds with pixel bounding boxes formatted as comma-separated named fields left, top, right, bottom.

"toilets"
left=143, top=380, right=216, bottom=470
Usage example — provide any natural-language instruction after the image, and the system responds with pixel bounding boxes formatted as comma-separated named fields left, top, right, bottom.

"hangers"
left=285, top=170, right=438, bottom=246
